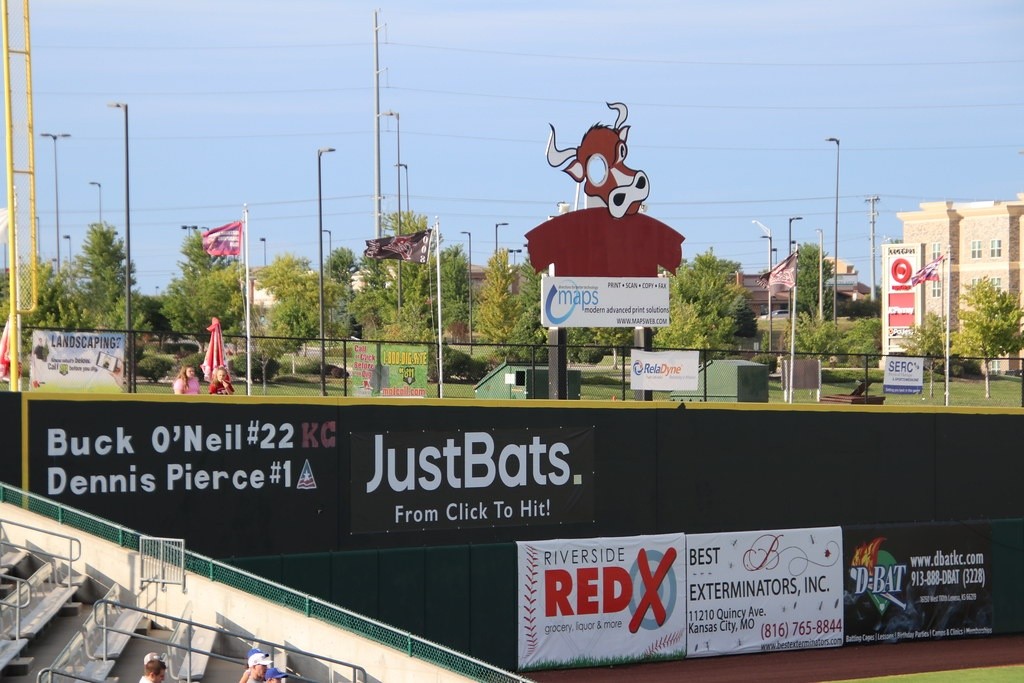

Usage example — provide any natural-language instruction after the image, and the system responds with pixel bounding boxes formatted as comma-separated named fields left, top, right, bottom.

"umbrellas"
left=201, top=316, right=225, bottom=383
left=0, top=314, right=23, bottom=383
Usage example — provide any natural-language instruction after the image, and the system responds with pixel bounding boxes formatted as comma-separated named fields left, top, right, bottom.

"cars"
left=769, top=310, right=788, bottom=318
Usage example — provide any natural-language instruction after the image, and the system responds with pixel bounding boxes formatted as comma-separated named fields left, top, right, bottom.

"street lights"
left=62, top=233, right=71, bottom=273
left=761, top=235, right=774, bottom=355
left=392, top=163, right=410, bottom=215
left=321, top=229, right=332, bottom=279
left=786, top=217, right=803, bottom=324
left=383, top=112, right=403, bottom=318
left=107, top=101, right=133, bottom=396
left=89, top=181, right=102, bottom=224
left=317, top=149, right=335, bottom=394
left=754, top=219, right=771, bottom=311
left=40, top=131, right=72, bottom=277
left=824, top=138, right=841, bottom=325
left=495, top=222, right=509, bottom=256
left=460, top=230, right=475, bottom=357
left=507, top=249, right=524, bottom=266
left=180, top=225, right=198, bottom=237
left=814, top=229, right=823, bottom=322
left=260, top=238, right=267, bottom=266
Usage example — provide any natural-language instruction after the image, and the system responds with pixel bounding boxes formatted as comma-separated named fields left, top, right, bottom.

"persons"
left=208, top=365, right=234, bottom=396
left=140, top=652, right=166, bottom=683
left=173, top=365, right=202, bottom=395
left=238, top=648, right=283, bottom=683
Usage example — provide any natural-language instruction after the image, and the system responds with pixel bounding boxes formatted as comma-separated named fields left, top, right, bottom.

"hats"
left=248, top=653, right=274, bottom=668
left=264, top=667, right=288, bottom=681
left=247, top=648, right=269, bottom=660
left=143, top=652, right=165, bottom=665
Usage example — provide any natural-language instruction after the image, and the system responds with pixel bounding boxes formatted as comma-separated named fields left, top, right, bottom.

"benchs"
left=160, top=601, right=218, bottom=679
left=254, top=640, right=287, bottom=683
left=0, top=525, right=144, bottom=683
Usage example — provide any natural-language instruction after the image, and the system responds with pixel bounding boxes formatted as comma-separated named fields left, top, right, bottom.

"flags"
left=363, top=228, right=434, bottom=265
left=202, top=221, right=243, bottom=257
left=756, top=249, right=798, bottom=299
left=911, top=254, right=944, bottom=287
